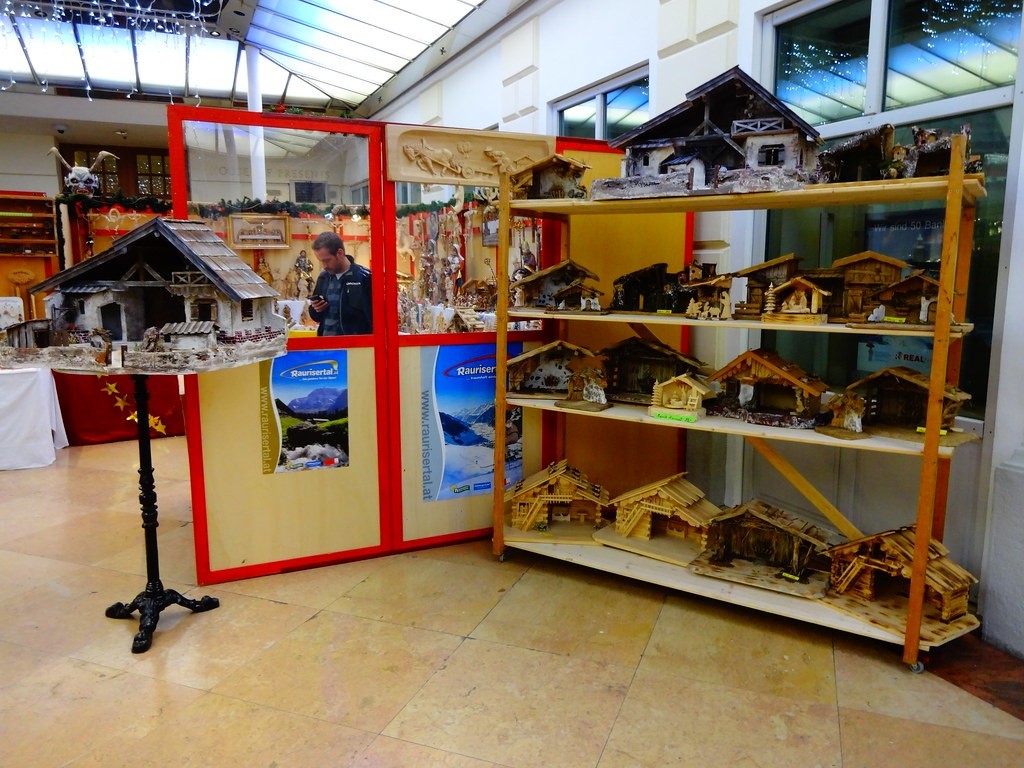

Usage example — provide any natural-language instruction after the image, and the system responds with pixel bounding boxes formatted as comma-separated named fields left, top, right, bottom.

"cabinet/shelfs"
left=0, top=195, right=59, bottom=258
left=490, top=133, right=990, bottom=672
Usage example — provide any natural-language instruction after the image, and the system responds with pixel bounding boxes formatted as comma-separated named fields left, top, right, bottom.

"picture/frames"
left=226, top=213, right=291, bottom=250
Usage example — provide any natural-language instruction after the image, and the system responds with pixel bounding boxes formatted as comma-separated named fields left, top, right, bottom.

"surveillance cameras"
left=55, top=125, right=66, bottom=134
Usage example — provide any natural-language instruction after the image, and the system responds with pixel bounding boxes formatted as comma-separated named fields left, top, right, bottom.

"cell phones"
left=310, top=295, right=322, bottom=303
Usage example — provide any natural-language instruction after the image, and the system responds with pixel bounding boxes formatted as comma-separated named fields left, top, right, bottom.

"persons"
left=294, top=250, right=315, bottom=299
left=257, top=256, right=273, bottom=287
left=483, top=147, right=515, bottom=175
left=308, top=231, right=372, bottom=336
